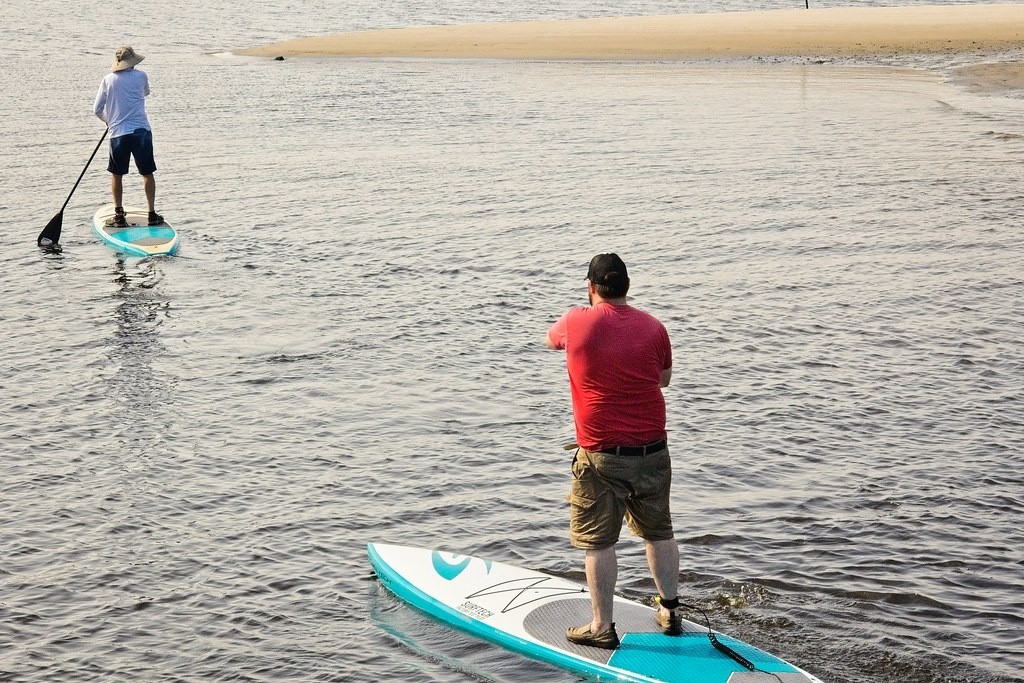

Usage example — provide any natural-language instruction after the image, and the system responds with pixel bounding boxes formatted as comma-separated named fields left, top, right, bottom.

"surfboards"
left=366, top=542, right=824, bottom=683
left=93, top=202, right=180, bottom=258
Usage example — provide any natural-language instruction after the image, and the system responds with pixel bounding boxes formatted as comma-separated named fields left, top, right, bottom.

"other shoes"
left=105, top=214, right=126, bottom=228
left=148, top=215, right=164, bottom=226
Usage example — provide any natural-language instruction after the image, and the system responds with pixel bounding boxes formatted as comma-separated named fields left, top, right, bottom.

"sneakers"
left=655, top=610, right=683, bottom=635
left=566, top=622, right=620, bottom=650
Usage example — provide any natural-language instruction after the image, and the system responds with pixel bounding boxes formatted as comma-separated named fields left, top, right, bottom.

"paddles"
left=37, top=127, right=109, bottom=246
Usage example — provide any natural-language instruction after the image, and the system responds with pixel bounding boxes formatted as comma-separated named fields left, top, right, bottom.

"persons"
left=93, top=47, right=164, bottom=227
left=546, top=254, right=682, bottom=648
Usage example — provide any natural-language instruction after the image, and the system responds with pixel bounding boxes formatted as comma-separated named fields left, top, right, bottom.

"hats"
left=111, top=46, right=145, bottom=73
left=582, top=253, right=629, bottom=288
